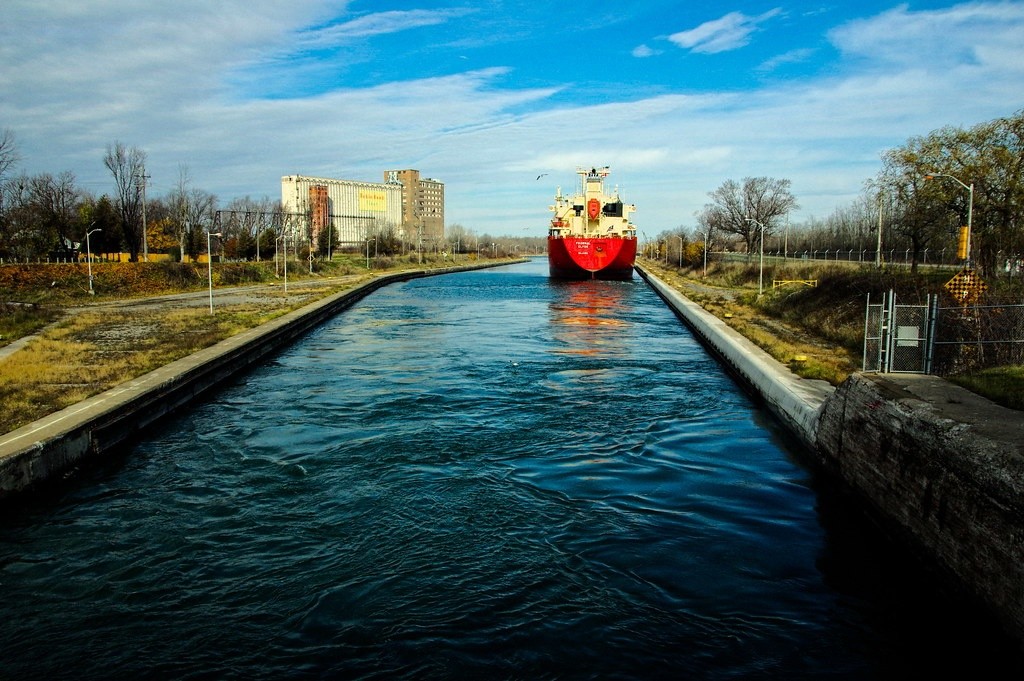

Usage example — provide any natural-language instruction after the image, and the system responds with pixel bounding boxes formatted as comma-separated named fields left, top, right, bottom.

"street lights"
left=419, top=241, right=425, bottom=265
left=367, top=239, right=375, bottom=269
left=454, top=242, right=459, bottom=261
left=745, top=218, right=763, bottom=295
left=661, top=238, right=668, bottom=265
left=536, top=240, right=536, bottom=255
left=784, top=203, right=800, bottom=258
left=276, top=235, right=286, bottom=278
left=515, top=245, right=519, bottom=256
left=674, top=235, right=682, bottom=269
left=647, top=242, right=653, bottom=260
left=478, top=243, right=484, bottom=260
left=925, top=172, right=975, bottom=313
left=640, top=244, right=644, bottom=257
left=496, top=244, right=500, bottom=258
left=510, top=244, right=514, bottom=253
left=693, top=229, right=707, bottom=276
left=207, top=231, right=223, bottom=314
left=86, top=229, right=103, bottom=295
left=644, top=243, right=648, bottom=258
left=409, top=242, right=412, bottom=257
left=877, top=186, right=899, bottom=266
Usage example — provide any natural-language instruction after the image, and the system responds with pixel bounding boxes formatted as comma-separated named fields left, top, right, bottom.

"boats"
left=546, top=165, right=638, bottom=281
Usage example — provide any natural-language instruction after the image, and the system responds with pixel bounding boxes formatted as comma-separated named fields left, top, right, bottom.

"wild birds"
left=536, top=173, right=549, bottom=181
left=508, top=359, right=520, bottom=369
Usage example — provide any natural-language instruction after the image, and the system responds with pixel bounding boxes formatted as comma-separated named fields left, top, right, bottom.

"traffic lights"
left=957, top=226, right=968, bottom=259
